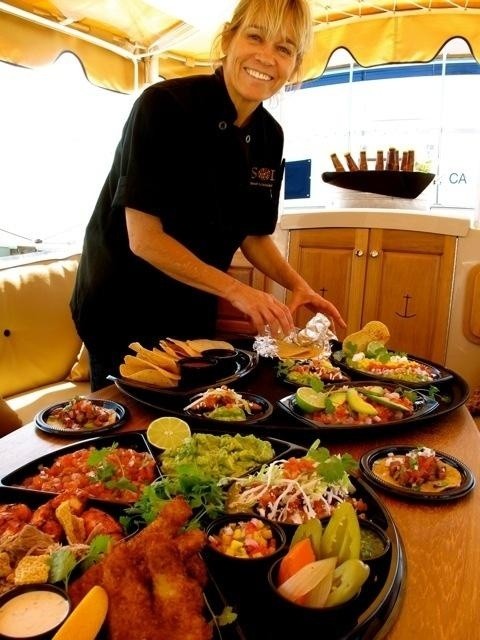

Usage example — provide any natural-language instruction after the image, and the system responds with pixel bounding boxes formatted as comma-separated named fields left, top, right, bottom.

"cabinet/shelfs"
left=277, top=205, right=471, bottom=376
left=217, top=249, right=267, bottom=341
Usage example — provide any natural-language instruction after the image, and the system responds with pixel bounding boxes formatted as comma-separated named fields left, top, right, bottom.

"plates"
left=109, top=349, right=259, bottom=405
left=339, top=351, right=443, bottom=384
left=359, top=439, right=476, bottom=509
left=111, top=339, right=470, bottom=431
left=40, top=397, right=126, bottom=437
left=2, top=425, right=407, bottom=640
left=183, top=390, right=274, bottom=425
left=278, top=382, right=440, bottom=430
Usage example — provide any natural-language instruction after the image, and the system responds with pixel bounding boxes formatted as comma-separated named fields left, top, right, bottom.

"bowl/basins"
left=321, top=170, right=436, bottom=201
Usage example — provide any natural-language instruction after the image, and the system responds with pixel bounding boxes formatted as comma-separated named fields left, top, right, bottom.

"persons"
left=67, top=0, right=347, bottom=396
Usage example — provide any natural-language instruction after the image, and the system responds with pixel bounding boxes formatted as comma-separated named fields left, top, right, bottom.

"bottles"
left=330, top=148, right=414, bottom=171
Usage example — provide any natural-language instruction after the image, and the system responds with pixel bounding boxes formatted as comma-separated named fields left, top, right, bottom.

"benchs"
left=0, top=257, right=94, bottom=444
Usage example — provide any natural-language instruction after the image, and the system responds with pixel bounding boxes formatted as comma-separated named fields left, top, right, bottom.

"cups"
left=203, top=344, right=241, bottom=378
left=176, top=355, right=212, bottom=384
left=212, top=512, right=284, bottom=581
left=266, top=557, right=362, bottom=629
left=315, top=515, right=391, bottom=569
left=0, top=580, right=70, bottom=640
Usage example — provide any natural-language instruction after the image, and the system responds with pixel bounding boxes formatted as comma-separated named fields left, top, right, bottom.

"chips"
left=119, top=336, right=320, bottom=387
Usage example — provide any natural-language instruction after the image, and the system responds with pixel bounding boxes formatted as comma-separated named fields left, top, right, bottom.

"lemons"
left=296, top=387, right=327, bottom=412
left=367, top=341, right=389, bottom=364
left=147, top=416, right=192, bottom=449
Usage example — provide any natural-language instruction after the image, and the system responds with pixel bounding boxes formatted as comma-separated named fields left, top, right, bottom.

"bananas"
left=347, top=388, right=378, bottom=416
